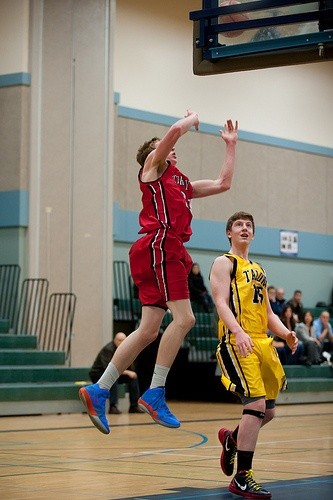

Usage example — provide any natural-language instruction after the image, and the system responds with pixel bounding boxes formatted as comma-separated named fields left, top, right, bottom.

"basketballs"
left=217, top=1, right=250, bottom=37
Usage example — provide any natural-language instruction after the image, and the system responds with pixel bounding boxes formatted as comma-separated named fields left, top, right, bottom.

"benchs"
left=159, top=310, right=333, bottom=392
left=113, top=297, right=143, bottom=310
left=0, top=316, right=126, bottom=400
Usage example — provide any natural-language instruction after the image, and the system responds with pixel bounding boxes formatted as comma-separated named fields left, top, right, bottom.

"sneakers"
left=218, top=427, right=238, bottom=476
left=228, top=469, right=272, bottom=500
left=137, top=385, right=182, bottom=429
left=78, top=384, right=111, bottom=434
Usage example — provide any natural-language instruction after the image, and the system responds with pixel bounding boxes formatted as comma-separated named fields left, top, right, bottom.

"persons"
left=187, top=263, right=213, bottom=314
left=209, top=210, right=299, bottom=500
left=79, top=108, right=238, bottom=436
left=267, top=286, right=333, bottom=367
left=88, top=331, right=145, bottom=414
